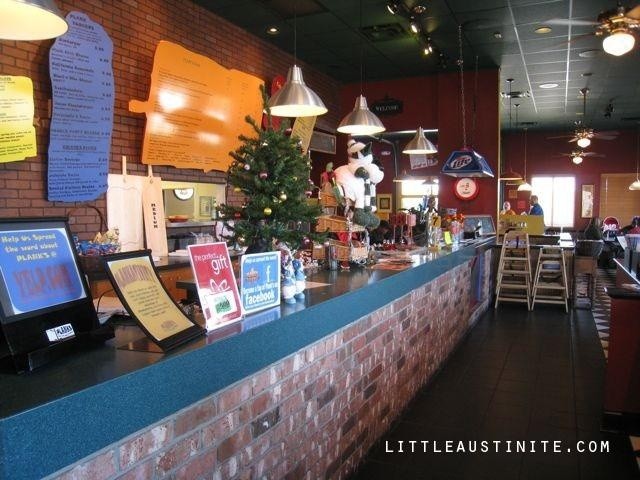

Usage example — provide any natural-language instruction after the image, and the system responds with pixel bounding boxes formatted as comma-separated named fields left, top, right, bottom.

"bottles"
left=451, top=218, right=458, bottom=244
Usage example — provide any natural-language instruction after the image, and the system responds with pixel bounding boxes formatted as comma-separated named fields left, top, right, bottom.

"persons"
left=528, top=195, right=543, bottom=215
left=629, top=217, right=640, bottom=234
left=584, top=217, right=601, bottom=240
left=501, top=201, right=516, bottom=215
left=370, top=220, right=389, bottom=244
left=427, top=195, right=437, bottom=213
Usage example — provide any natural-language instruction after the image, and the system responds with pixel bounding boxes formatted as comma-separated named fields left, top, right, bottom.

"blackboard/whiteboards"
left=464, top=214, right=496, bottom=235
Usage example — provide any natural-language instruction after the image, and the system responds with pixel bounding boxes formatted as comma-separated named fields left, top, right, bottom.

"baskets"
left=67, top=204, right=106, bottom=272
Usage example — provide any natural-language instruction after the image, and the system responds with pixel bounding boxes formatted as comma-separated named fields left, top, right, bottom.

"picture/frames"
left=581, top=183, right=595, bottom=219
left=377, top=193, right=392, bottom=213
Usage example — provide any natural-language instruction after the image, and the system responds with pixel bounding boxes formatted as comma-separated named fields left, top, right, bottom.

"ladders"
left=494, top=230, right=533, bottom=311
left=531, top=246, right=568, bottom=313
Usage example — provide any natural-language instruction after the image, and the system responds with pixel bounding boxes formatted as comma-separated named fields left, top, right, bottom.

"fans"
left=552, top=0, right=640, bottom=64
left=546, top=88, right=620, bottom=148
left=551, top=146, right=606, bottom=165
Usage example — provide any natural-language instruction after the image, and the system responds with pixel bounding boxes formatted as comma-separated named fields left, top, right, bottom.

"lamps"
left=400, top=119, right=439, bottom=154
left=602, top=30, right=636, bottom=58
left=336, top=0, right=386, bottom=136
left=572, top=156, right=584, bottom=165
left=263, top=1, right=328, bottom=118
left=501, top=77, right=534, bottom=193
left=0, top=0, right=70, bottom=40
left=387, top=1, right=447, bottom=70
left=441, top=23, right=496, bottom=179
left=577, top=138, right=590, bottom=148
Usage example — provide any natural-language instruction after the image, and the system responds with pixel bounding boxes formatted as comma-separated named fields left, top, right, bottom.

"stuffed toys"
left=323, top=138, right=384, bottom=228
left=278, top=241, right=306, bottom=304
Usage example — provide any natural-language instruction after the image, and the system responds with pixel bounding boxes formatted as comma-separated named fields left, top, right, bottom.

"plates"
left=168, top=215, right=188, bottom=222
